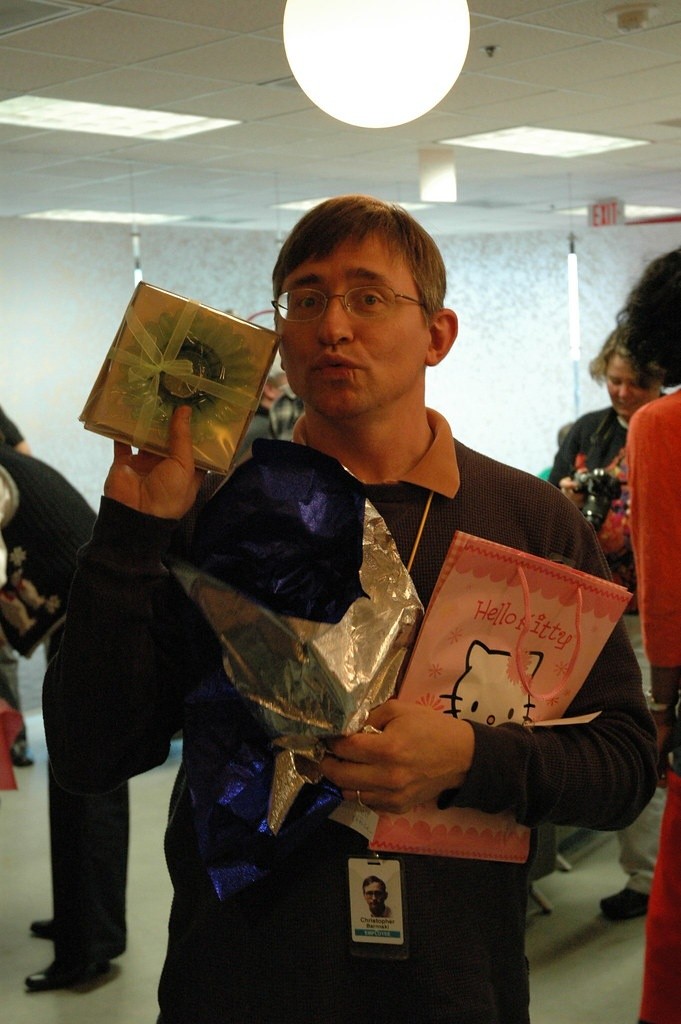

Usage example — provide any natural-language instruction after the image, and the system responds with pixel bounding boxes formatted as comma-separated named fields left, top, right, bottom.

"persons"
left=362, top=876, right=392, bottom=917
left=0, top=196, right=681, bottom=1024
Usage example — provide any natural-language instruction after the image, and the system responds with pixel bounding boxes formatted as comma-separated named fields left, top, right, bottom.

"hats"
left=268, top=372, right=288, bottom=388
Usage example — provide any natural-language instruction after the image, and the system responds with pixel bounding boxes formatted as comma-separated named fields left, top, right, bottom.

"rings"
left=356, top=790, right=364, bottom=807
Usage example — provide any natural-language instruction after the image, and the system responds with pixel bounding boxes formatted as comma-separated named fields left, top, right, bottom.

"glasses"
left=271, top=285, right=422, bottom=322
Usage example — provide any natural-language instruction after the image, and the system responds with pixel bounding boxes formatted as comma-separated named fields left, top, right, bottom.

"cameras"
left=574, top=468, right=623, bottom=532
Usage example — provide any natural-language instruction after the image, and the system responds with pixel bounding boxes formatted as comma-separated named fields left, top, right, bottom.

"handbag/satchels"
left=368, top=530, right=634, bottom=863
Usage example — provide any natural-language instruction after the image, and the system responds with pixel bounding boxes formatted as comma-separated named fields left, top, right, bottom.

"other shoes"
left=31, top=921, right=54, bottom=939
left=600, top=887, right=649, bottom=922
left=26, top=959, right=111, bottom=990
